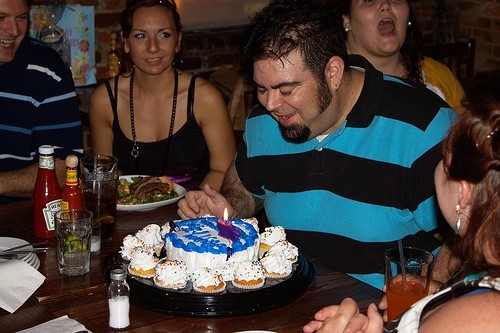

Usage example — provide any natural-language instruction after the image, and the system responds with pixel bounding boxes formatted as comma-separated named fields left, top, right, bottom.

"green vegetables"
left=118, top=175, right=179, bottom=204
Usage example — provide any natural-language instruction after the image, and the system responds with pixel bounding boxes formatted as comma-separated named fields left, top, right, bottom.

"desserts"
left=120, top=214, right=299, bottom=293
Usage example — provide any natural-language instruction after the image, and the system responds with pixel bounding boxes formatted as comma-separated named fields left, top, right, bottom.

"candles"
left=216, top=206, right=237, bottom=240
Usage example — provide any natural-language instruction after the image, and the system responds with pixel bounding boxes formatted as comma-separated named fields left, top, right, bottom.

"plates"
left=0, top=237, right=40, bottom=270
left=116, top=175, right=187, bottom=211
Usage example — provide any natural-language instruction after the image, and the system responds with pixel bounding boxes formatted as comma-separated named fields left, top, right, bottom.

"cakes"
left=164, top=216, right=260, bottom=282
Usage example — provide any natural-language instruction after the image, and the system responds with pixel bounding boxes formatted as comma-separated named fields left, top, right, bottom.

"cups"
left=55, top=209, right=93, bottom=276
left=384, top=246, right=435, bottom=333
left=79, top=172, right=119, bottom=245
left=81, top=154, right=118, bottom=172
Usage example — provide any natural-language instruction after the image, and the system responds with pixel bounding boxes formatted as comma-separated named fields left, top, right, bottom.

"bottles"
left=90, top=220, right=101, bottom=255
left=61, top=155, right=83, bottom=221
left=107, top=269, right=131, bottom=331
left=33, top=145, right=60, bottom=238
left=109, top=32, right=121, bottom=77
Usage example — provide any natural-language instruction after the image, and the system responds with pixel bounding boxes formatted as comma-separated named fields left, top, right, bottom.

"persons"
left=342, top=0, right=468, bottom=114
left=177, top=0, right=465, bottom=290
left=0, top=0, right=84, bottom=198
left=304, top=71, right=500, bottom=333
left=91, top=0, right=236, bottom=193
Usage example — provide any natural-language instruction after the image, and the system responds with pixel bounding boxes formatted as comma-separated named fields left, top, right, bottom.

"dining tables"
left=0, top=193, right=386, bottom=333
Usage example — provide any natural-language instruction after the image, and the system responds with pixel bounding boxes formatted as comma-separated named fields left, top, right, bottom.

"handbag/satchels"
left=209, top=67, right=255, bottom=131
